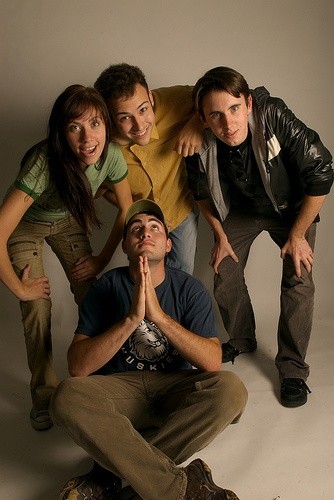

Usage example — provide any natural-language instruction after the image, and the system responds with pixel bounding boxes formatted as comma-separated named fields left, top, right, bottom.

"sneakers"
left=58, top=461, right=122, bottom=500
left=222, top=342, right=257, bottom=365
left=30, top=407, right=53, bottom=432
left=184, top=458, right=240, bottom=500
left=280, top=378, right=311, bottom=408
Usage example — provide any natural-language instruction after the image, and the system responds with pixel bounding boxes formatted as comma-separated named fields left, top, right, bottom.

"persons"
left=1, top=85, right=133, bottom=431
left=47, top=199, right=248, bottom=500
left=185, top=66, right=333, bottom=409
left=95, top=64, right=204, bottom=275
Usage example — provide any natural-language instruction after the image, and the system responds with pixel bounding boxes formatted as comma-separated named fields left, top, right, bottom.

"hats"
left=123, top=198, right=169, bottom=238
